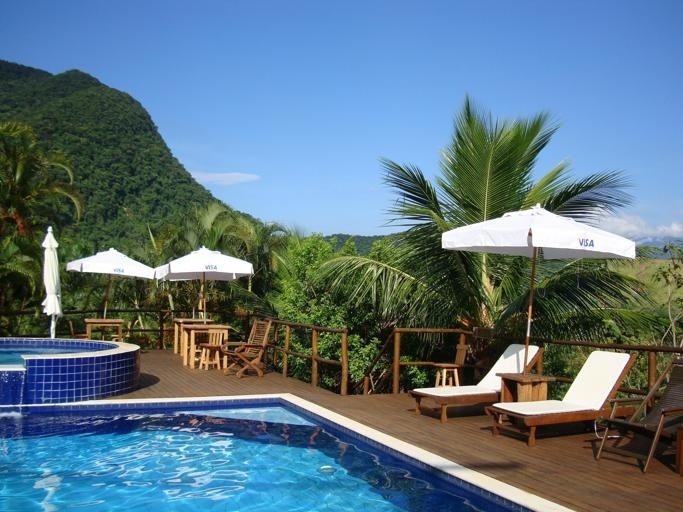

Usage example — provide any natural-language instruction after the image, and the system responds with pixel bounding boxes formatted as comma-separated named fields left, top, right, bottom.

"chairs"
left=110, top=319, right=140, bottom=342
left=594, top=353, right=683, bottom=473
left=196, top=318, right=273, bottom=378
left=67, top=320, right=87, bottom=340
left=484, top=350, right=637, bottom=447
left=407, top=341, right=545, bottom=423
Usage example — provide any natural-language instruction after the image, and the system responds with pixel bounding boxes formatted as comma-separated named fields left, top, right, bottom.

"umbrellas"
left=66, top=246, right=156, bottom=341
left=40, top=226, right=64, bottom=339
left=153, top=246, right=255, bottom=323
left=438, top=202, right=637, bottom=372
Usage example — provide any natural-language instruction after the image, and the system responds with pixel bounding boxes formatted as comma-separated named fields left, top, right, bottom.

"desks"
left=83, top=317, right=123, bottom=342
left=173, top=318, right=232, bottom=369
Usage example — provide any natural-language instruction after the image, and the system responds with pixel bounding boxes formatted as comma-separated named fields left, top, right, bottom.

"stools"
left=433, top=363, right=460, bottom=387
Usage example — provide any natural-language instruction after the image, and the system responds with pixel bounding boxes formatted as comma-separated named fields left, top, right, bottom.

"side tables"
left=496, top=372, right=557, bottom=428
